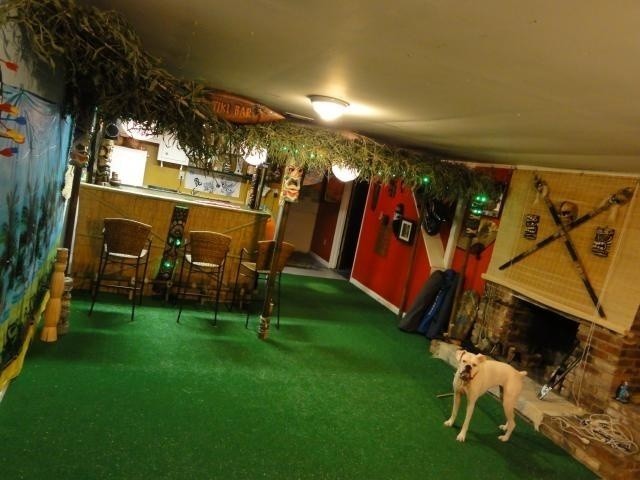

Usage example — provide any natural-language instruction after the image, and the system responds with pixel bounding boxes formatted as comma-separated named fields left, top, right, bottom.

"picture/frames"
left=397, top=217, right=416, bottom=245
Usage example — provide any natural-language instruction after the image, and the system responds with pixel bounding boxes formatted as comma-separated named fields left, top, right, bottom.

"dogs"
left=443, top=349, right=528, bottom=442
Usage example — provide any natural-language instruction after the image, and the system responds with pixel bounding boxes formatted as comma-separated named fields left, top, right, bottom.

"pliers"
left=536, top=341, right=593, bottom=400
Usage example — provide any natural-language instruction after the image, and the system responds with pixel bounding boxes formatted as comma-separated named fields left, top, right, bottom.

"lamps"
left=310, top=96, right=351, bottom=123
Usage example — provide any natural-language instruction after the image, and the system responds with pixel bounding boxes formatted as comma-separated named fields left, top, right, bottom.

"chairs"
left=86, top=218, right=153, bottom=322
left=173, top=230, right=232, bottom=327
left=229, top=240, right=296, bottom=330
